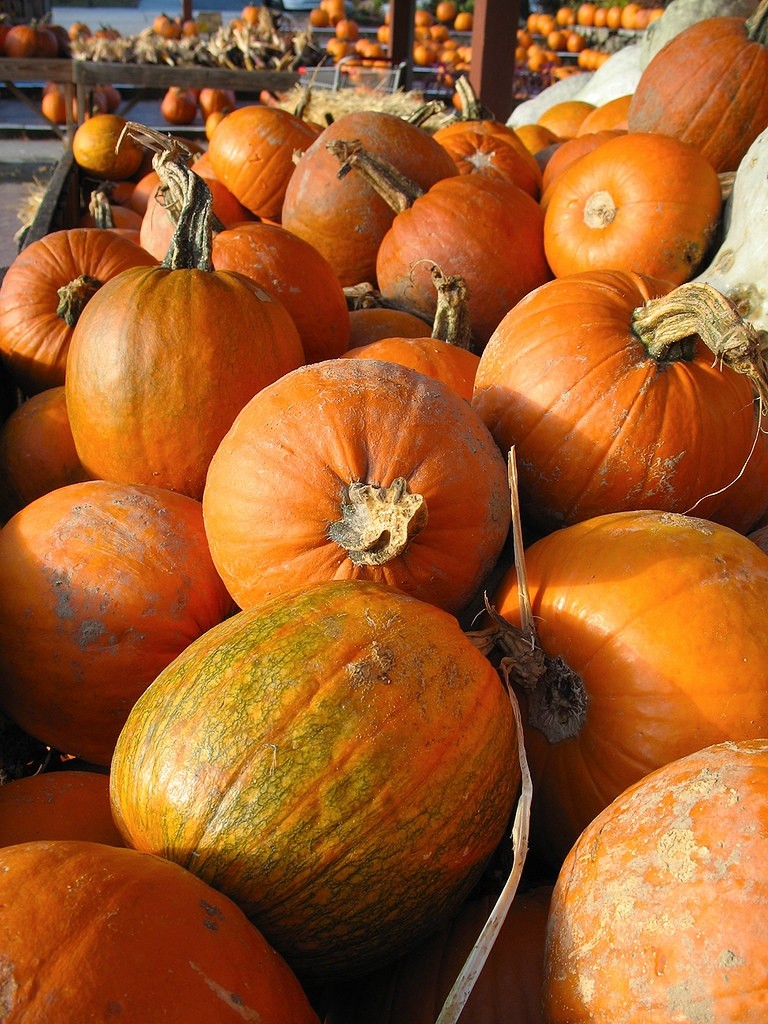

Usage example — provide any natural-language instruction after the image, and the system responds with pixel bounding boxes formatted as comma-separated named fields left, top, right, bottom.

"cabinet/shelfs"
left=0, top=57, right=74, bottom=151
left=74, top=48, right=334, bottom=137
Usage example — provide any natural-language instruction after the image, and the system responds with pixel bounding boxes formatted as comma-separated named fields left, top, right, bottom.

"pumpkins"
left=0, top=0, right=768, bottom=1024
